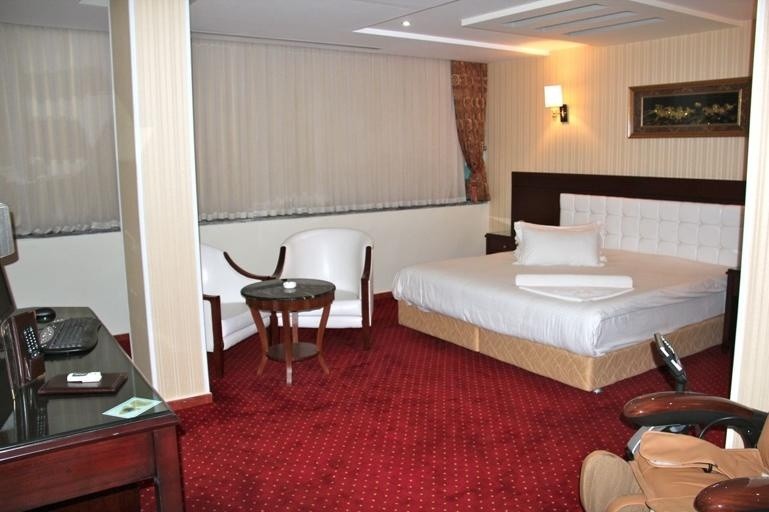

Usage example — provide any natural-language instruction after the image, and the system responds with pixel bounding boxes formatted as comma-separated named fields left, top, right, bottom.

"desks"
left=240, top=277, right=337, bottom=386
left=0, top=307, right=184, bottom=512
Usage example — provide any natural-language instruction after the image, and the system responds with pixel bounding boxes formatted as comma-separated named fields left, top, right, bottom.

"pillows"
left=514, top=215, right=602, bottom=268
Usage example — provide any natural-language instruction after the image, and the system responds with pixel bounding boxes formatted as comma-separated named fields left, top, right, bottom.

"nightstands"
left=723, top=268, right=742, bottom=350
left=485, top=231, right=517, bottom=254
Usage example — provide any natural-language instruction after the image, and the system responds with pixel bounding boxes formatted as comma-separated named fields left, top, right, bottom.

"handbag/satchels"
left=603, top=429, right=766, bottom=512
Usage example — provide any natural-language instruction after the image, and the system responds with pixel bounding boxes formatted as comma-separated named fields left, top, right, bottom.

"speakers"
left=0, top=203, right=16, bottom=259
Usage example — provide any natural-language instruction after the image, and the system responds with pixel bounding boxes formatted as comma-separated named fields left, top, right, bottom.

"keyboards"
left=38, top=317, right=101, bottom=354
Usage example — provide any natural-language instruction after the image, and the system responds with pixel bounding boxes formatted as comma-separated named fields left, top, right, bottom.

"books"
left=36, top=371, right=129, bottom=394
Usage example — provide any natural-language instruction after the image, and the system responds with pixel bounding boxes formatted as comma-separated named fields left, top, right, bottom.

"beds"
left=394, top=170, right=745, bottom=395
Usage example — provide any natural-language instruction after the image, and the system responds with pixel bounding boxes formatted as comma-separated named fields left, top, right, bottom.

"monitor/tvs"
left=656, top=332, right=683, bottom=372
left=0, top=264, right=16, bottom=326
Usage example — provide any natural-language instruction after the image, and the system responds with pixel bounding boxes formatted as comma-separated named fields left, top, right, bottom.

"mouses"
left=35, top=307, right=56, bottom=323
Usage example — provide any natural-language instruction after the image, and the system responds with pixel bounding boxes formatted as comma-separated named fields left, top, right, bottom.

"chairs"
left=579, top=391, right=769, bottom=512
left=198, top=227, right=374, bottom=377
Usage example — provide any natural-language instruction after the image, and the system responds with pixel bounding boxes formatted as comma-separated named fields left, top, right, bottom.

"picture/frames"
left=628, top=76, right=752, bottom=137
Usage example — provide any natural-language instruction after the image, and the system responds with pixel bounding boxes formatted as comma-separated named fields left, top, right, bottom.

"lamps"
left=543, top=85, right=569, bottom=124
